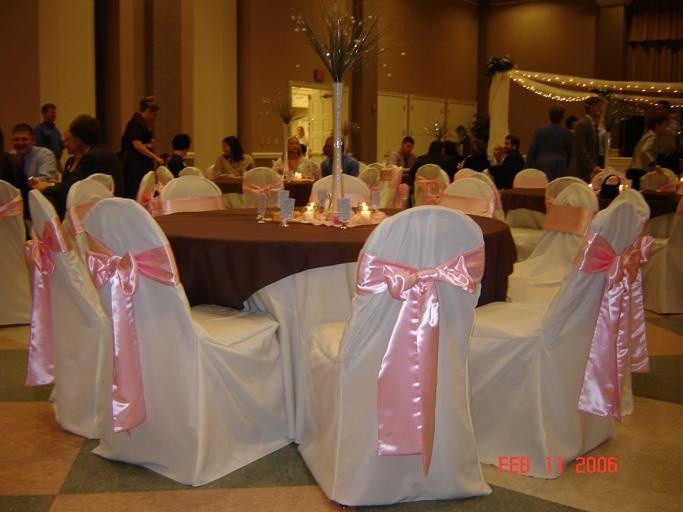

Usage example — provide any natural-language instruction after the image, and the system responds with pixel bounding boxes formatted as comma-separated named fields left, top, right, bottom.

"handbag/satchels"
left=600, top=174, right=621, bottom=198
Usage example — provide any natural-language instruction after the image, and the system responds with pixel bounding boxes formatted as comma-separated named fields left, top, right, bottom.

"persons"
left=0, top=129, right=27, bottom=222
left=118, top=95, right=166, bottom=199
left=457, top=136, right=492, bottom=180
left=46, top=116, right=118, bottom=185
left=571, top=95, right=607, bottom=184
left=6, top=123, right=55, bottom=190
left=563, top=115, right=577, bottom=133
left=387, top=136, right=419, bottom=177
left=623, top=114, right=665, bottom=189
left=617, top=113, right=646, bottom=158
left=501, top=134, right=525, bottom=178
left=165, top=132, right=189, bottom=178
left=610, top=109, right=623, bottom=149
left=650, top=100, right=681, bottom=172
left=523, top=103, right=575, bottom=181
left=484, top=143, right=515, bottom=189
left=30, top=120, right=117, bottom=227
left=596, top=118, right=611, bottom=169
left=270, top=135, right=321, bottom=184
left=320, top=137, right=361, bottom=182
left=212, top=135, right=255, bottom=195
left=449, top=124, right=472, bottom=170
left=32, top=102, right=68, bottom=170
left=405, top=140, right=456, bottom=193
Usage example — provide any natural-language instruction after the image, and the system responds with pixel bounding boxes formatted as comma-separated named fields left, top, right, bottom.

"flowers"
left=425, top=119, right=449, bottom=141
left=483, top=53, right=516, bottom=87
left=269, top=85, right=309, bottom=123
left=289, top=3, right=395, bottom=81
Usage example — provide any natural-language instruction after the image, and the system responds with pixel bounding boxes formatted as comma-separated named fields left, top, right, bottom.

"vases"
left=329, top=82, right=347, bottom=208
left=284, top=124, right=292, bottom=173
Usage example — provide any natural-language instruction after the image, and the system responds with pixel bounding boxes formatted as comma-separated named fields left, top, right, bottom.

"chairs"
left=605, top=188, right=650, bottom=216
left=309, top=174, right=371, bottom=210
left=135, top=170, right=155, bottom=206
left=467, top=200, right=648, bottom=477
left=508, top=185, right=600, bottom=301
left=160, top=177, right=224, bottom=217
left=506, top=168, right=549, bottom=262
left=243, top=167, right=284, bottom=209
left=83, top=196, right=289, bottom=486
left=646, top=182, right=683, bottom=314
left=443, top=177, right=496, bottom=216
left=0, top=179, right=32, bottom=328
left=412, top=163, right=451, bottom=207
left=179, top=165, right=204, bottom=178
left=359, top=167, right=379, bottom=187
left=66, top=179, right=115, bottom=259
left=157, top=165, right=176, bottom=186
left=295, top=206, right=490, bottom=505
left=378, top=163, right=400, bottom=210
left=26, top=190, right=115, bottom=438
left=468, top=173, right=505, bottom=218
left=86, top=172, right=114, bottom=192
left=452, top=169, right=478, bottom=182
left=548, top=176, right=592, bottom=213
